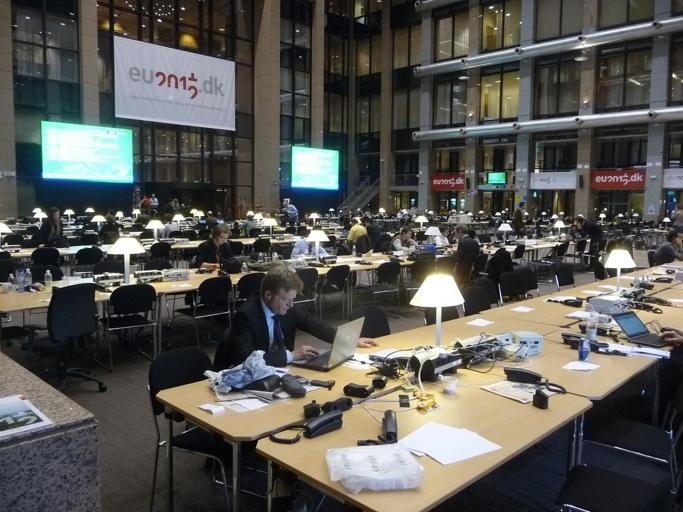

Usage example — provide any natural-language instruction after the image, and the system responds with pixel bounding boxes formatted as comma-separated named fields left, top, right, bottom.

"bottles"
left=273, top=252, right=278, bottom=261
left=586, top=316, right=598, bottom=340
left=352, top=244, right=356, bottom=258
left=3, top=242, right=9, bottom=251
left=633, top=272, right=640, bottom=289
left=241, top=262, right=247, bottom=276
left=258, top=252, right=263, bottom=264
left=44, top=270, right=52, bottom=292
left=6, top=268, right=32, bottom=295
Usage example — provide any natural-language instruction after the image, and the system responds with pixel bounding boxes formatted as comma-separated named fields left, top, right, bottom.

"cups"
left=643, top=274, right=649, bottom=281
left=441, top=375, right=457, bottom=395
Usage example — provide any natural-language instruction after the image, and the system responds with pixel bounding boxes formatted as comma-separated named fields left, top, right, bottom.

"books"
left=0, top=395, right=57, bottom=442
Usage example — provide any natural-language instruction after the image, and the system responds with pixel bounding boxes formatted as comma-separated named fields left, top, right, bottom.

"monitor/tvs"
left=487, top=171, right=507, bottom=185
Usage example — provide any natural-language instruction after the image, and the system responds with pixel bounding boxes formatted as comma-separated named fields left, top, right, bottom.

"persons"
left=42, top=194, right=683, bottom=493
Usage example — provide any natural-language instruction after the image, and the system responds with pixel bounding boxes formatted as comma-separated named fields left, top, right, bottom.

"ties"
left=271, top=314, right=285, bottom=349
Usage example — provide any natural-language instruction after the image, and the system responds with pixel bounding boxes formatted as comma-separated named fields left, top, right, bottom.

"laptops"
left=611, top=310, right=672, bottom=347
left=294, top=316, right=365, bottom=371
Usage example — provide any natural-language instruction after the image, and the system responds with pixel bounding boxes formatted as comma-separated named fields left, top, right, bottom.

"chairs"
left=0, top=199, right=682, bottom=511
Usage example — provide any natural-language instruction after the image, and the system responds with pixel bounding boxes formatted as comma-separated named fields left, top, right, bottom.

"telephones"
left=504, top=367, right=542, bottom=383
left=640, top=277, right=673, bottom=290
left=303, top=410, right=342, bottom=438
left=25, top=285, right=40, bottom=292
left=218, top=270, right=230, bottom=277
left=378, top=410, right=397, bottom=443
left=562, top=324, right=607, bottom=351
left=96, top=283, right=112, bottom=292
left=564, top=294, right=667, bottom=310
